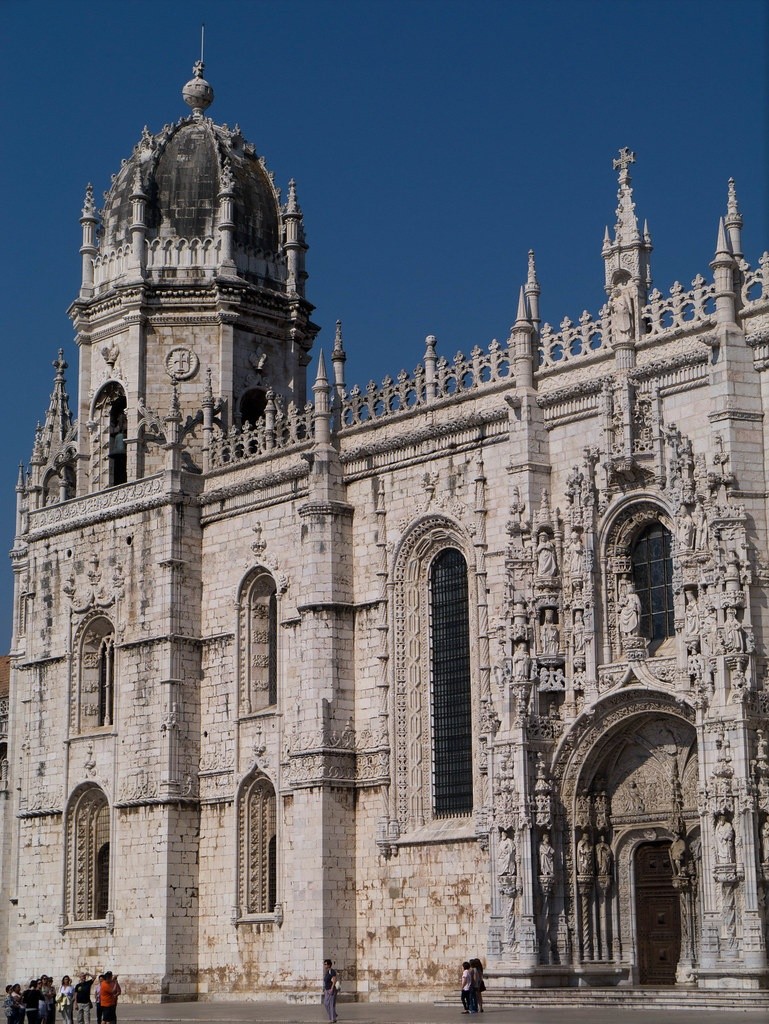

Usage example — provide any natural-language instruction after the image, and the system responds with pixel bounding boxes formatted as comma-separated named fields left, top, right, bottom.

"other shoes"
left=478, top=1009, right=483, bottom=1012
left=461, top=1010, right=469, bottom=1014
left=329, top=1020, right=337, bottom=1024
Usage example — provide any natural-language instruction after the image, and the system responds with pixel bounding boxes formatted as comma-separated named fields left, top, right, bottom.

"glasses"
left=42, top=978, right=47, bottom=980
left=324, top=964, right=328, bottom=965
left=37, top=982, right=42, bottom=985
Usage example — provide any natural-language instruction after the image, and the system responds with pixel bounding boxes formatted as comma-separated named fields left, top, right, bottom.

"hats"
left=77, top=972, right=86, bottom=977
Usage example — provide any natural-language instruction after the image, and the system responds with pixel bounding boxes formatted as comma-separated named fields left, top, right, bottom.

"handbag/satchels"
left=329, top=969, right=341, bottom=989
left=479, top=980, right=486, bottom=991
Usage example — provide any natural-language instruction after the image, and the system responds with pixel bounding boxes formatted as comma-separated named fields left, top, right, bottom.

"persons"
left=513, top=287, right=743, bottom=683
left=499, top=780, right=769, bottom=878
left=324, top=959, right=339, bottom=1023
left=460, top=958, right=485, bottom=1013
left=4, top=971, right=120, bottom=1024
